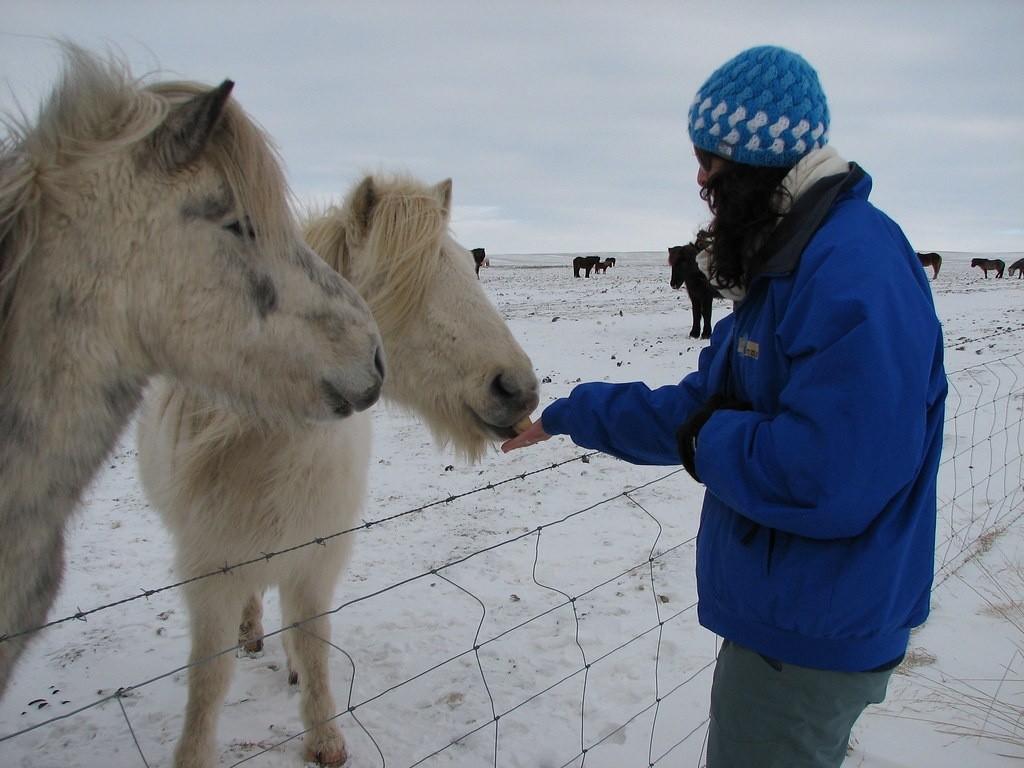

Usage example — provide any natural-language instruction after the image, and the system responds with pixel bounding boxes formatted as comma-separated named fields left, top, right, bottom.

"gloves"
left=675, top=396, right=755, bottom=483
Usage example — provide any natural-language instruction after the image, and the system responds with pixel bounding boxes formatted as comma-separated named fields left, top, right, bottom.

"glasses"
left=693, top=145, right=713, bottom=173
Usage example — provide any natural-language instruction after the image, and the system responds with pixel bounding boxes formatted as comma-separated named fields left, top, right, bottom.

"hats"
left=690, top=48, right=830, bottom=169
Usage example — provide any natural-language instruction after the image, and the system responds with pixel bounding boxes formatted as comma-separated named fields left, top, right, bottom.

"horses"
left=571, top=240, right=1024, bottom=341
left=133, top=168, right=541, bottom=768
left=1, top=30, right=386, bottom=716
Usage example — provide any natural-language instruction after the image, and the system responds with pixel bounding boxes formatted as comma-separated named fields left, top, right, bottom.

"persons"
left=501, top=45, right=949, bottom=768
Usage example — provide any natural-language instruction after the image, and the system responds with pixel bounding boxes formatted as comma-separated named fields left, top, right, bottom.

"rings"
left=526, top=441, right=532, bottom=445
